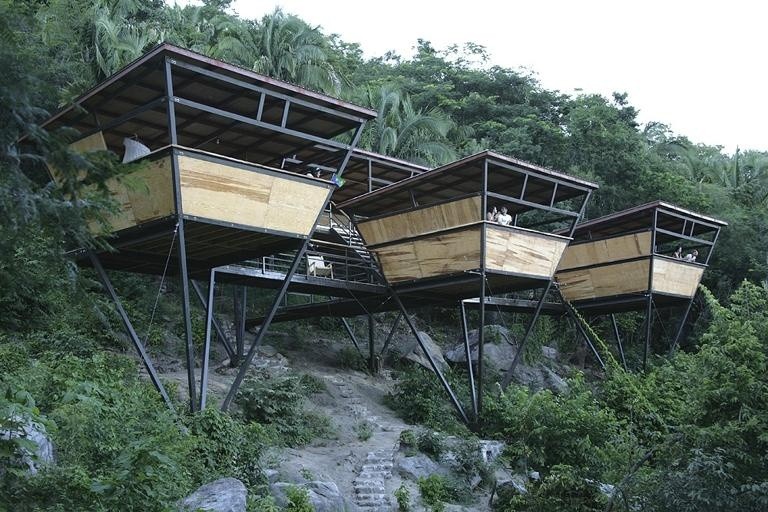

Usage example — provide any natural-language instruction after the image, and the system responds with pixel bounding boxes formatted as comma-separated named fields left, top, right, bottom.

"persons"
left=306, top=167, right=322, bottom=179
left=670, top=247, right=682, bottom=259
left=681, top=249, right=698, bottom=263
left=494, top=204, right=512, bottom=226
left=486, top=203, right=500, bottom=222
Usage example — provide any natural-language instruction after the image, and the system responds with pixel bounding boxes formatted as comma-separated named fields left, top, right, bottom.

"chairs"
left=305, top=254, right=334, bottom=281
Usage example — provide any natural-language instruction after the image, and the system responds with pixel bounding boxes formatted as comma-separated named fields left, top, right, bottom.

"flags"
left=330, top=172, right=346, bottom=189
